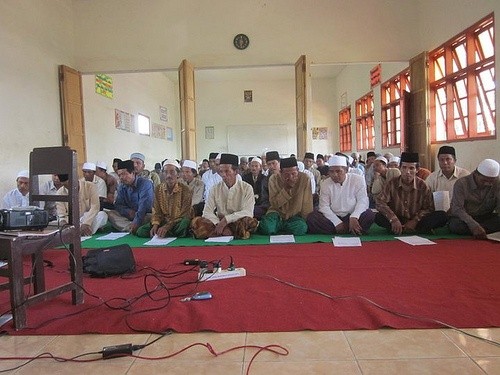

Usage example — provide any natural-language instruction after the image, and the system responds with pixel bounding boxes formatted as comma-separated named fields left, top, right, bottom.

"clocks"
left=234, top=33, right=250, bottom=50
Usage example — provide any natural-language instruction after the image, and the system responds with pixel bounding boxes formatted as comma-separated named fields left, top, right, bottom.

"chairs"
left=0, top=146, right=84, bottom=332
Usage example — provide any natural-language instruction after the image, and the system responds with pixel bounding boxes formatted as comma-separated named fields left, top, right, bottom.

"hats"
left=162, top=150, right=400, bottom=169
left=83, top=161, right=96, bottom=170
left=113, top=152, right=145, bottom=169
left=439, top=146, right=456, bottom=154
left=477, top=158, right=499, bottom=177
left=16, top=170, right=30, bottom=179
left=401, top=153, right=420, bottom=162
left=97, top=160, right=107, bottom=169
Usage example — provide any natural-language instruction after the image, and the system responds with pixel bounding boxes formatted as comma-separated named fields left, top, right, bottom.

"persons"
left=0, top=145, right=500, bottom=239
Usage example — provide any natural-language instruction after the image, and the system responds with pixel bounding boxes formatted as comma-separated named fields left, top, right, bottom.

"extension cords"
left=198, top=267, right=247, bottom=281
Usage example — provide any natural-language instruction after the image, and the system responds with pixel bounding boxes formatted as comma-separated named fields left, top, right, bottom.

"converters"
left=103, top=343, right=132, bottom=356
left=185, top=260, right=202, bottom=265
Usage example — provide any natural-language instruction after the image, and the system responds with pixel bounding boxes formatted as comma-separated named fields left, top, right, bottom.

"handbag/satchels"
left=84, top=244, right=136, bottom=277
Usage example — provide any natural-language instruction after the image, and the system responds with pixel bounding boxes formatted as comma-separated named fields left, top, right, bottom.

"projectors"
left=0, top=209, right=52, bottom=231
